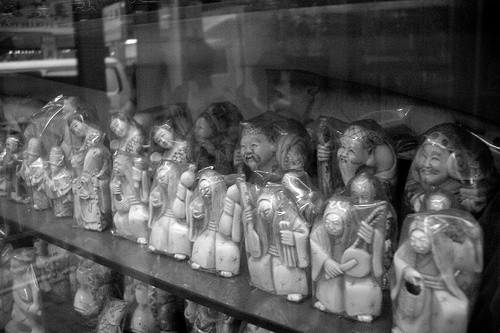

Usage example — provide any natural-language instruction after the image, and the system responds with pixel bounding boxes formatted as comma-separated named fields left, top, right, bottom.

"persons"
left=0, top=86, right=499, bottom=332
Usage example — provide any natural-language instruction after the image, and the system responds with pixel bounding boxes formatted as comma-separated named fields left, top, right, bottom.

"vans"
left=1, top=58, right=131, bottom=121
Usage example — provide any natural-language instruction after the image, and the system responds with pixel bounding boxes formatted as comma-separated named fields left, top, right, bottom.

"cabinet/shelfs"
left=1, top=0, right=499, bottom=333
